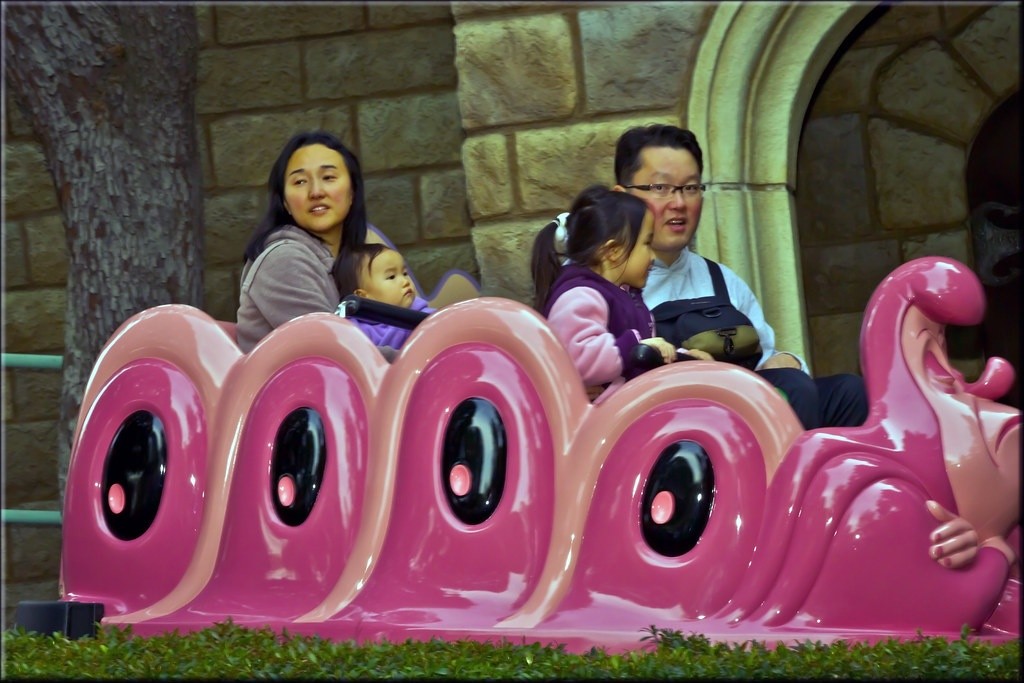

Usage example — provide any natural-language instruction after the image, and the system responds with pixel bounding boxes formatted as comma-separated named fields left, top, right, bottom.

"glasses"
left=623, top=183, right=706, bottom=199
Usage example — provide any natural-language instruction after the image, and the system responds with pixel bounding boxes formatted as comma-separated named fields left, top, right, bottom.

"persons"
left=236, top=130, right=401, bottom=365
left=562, top=123, right=869, bottom=431
left=332, top=243, right=438, bottom=351
left=530, top=184, right=716, bottom=404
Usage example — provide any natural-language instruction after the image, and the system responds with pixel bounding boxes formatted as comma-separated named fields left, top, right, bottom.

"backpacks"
left=650, top=255, right=764, bottom=371
